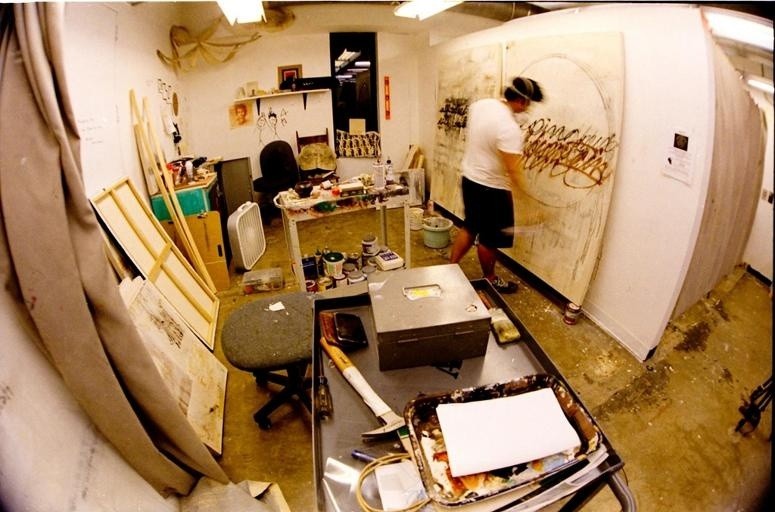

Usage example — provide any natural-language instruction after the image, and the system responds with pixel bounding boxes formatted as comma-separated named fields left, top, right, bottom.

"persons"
left=449, top=77, right=544, bottom=295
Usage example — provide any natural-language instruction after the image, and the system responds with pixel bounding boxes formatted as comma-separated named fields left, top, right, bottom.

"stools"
left=218, top=294, right=313, bottom=433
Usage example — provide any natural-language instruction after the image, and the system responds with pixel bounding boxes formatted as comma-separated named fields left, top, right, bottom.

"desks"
left=276, top=184, right=412, bottom=295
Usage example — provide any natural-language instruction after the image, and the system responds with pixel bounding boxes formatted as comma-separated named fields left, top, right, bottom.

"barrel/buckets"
left=407, top=208, right=424, bottom=232
left=322, top=254, right=346, bottom=278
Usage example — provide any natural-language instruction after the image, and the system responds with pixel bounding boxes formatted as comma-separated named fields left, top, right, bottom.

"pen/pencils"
left=351, top=449, right=392, bottom=467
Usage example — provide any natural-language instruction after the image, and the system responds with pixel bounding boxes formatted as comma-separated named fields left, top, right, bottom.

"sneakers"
left=481, top=276, right=518, bottom=294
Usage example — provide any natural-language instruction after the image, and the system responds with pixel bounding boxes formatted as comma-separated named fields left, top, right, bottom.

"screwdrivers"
left=313, top=346, right=334, bottom=418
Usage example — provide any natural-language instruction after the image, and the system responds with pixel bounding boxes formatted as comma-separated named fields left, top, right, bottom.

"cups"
left=294, top=180, right=314, bottom=199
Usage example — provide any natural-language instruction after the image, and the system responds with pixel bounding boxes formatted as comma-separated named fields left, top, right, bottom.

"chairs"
left=294, top=127, right=340, bottom=186
left=250, top=140, right=300, bottom=226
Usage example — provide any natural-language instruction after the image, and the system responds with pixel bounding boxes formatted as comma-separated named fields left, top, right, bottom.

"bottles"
left=372, top=154, right=395, bottom=191
left=563, top=302, right=583, bottom=327
left=303, top=235, right=404, bottom=294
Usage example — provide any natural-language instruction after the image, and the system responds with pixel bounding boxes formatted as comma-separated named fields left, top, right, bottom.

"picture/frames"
left=276, top=64, right=303, bottom=86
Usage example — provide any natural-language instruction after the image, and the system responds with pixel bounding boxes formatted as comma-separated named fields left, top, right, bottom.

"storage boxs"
left=365, top=264, right=493, bottom=372
